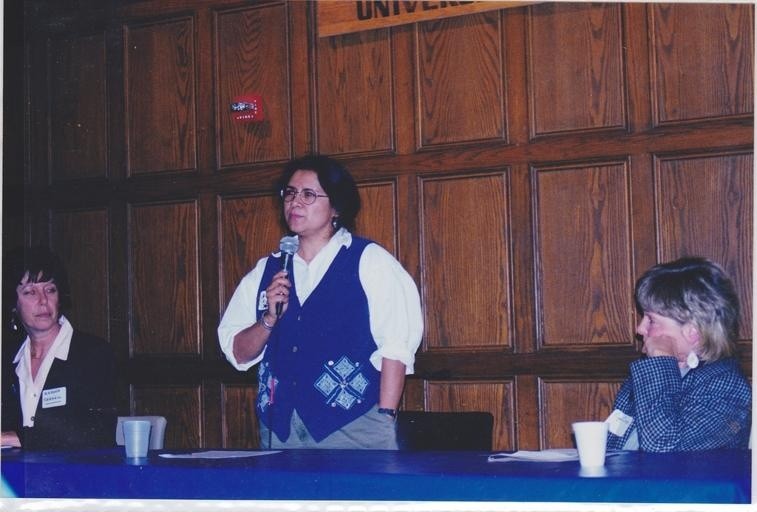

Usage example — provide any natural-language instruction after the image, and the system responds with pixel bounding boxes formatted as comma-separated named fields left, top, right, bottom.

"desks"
left=1, top=445, right=748, bottom=501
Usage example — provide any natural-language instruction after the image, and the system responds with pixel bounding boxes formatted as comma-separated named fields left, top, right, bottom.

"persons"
left=2, top=249, right=125, bottom=451
left=217, top=155, right=423, bottom=449
left=605, top=258, right=753, bottom=455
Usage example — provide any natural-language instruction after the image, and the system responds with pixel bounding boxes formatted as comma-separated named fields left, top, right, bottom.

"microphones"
left=276, top=235, right=300, bottom=321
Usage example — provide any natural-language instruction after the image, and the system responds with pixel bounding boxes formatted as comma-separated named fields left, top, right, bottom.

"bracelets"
left=260, top=310, right=275, bottom=330
left=378, top=407, right=398, bottom=419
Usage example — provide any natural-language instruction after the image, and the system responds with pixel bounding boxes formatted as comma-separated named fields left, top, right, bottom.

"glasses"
left=279, top=188, right=331, bottom=204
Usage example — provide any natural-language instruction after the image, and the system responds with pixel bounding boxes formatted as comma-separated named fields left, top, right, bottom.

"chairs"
left=392, top=409, right=493, bottom=452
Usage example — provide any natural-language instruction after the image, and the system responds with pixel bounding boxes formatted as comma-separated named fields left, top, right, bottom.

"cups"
left=570, top=421, right=609, bottom=468
left=121, top=420, right=151, bottom=458
